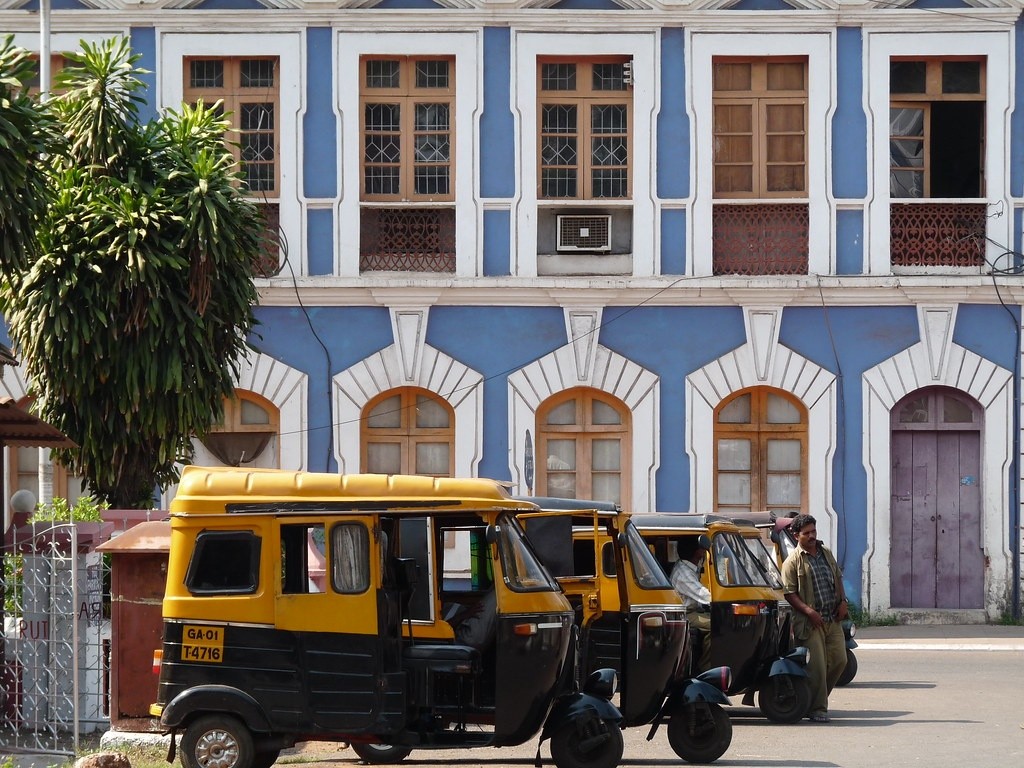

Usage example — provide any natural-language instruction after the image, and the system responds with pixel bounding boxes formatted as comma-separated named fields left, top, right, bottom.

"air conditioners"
left=556, top=214, right=612, bottom=252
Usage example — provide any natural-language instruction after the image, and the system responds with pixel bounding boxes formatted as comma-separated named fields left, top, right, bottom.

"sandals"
left=810, top=708, right=831, bottom=723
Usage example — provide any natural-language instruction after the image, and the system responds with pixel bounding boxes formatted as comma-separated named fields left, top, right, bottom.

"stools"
left=405, top=644, right=479, bottom=731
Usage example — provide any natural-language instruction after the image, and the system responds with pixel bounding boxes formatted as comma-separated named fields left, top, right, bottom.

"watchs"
left=844, top=598, right=848, bottom=603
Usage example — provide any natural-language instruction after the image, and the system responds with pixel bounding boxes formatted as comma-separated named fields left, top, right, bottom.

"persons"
left=453, top=589, right=497, bottom=644
left=780, top=514, right=848, bottom=723
left=671, top=536, right=713, bottom=671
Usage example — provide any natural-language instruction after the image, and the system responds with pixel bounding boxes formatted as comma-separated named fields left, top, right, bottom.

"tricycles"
left=396, top=495, right=733, bottom=762
left=573, top=512, right=814, bottom=723
left=148, top=465, right=624, bottom=767
left=646, top=510, right=860, bottom=688
